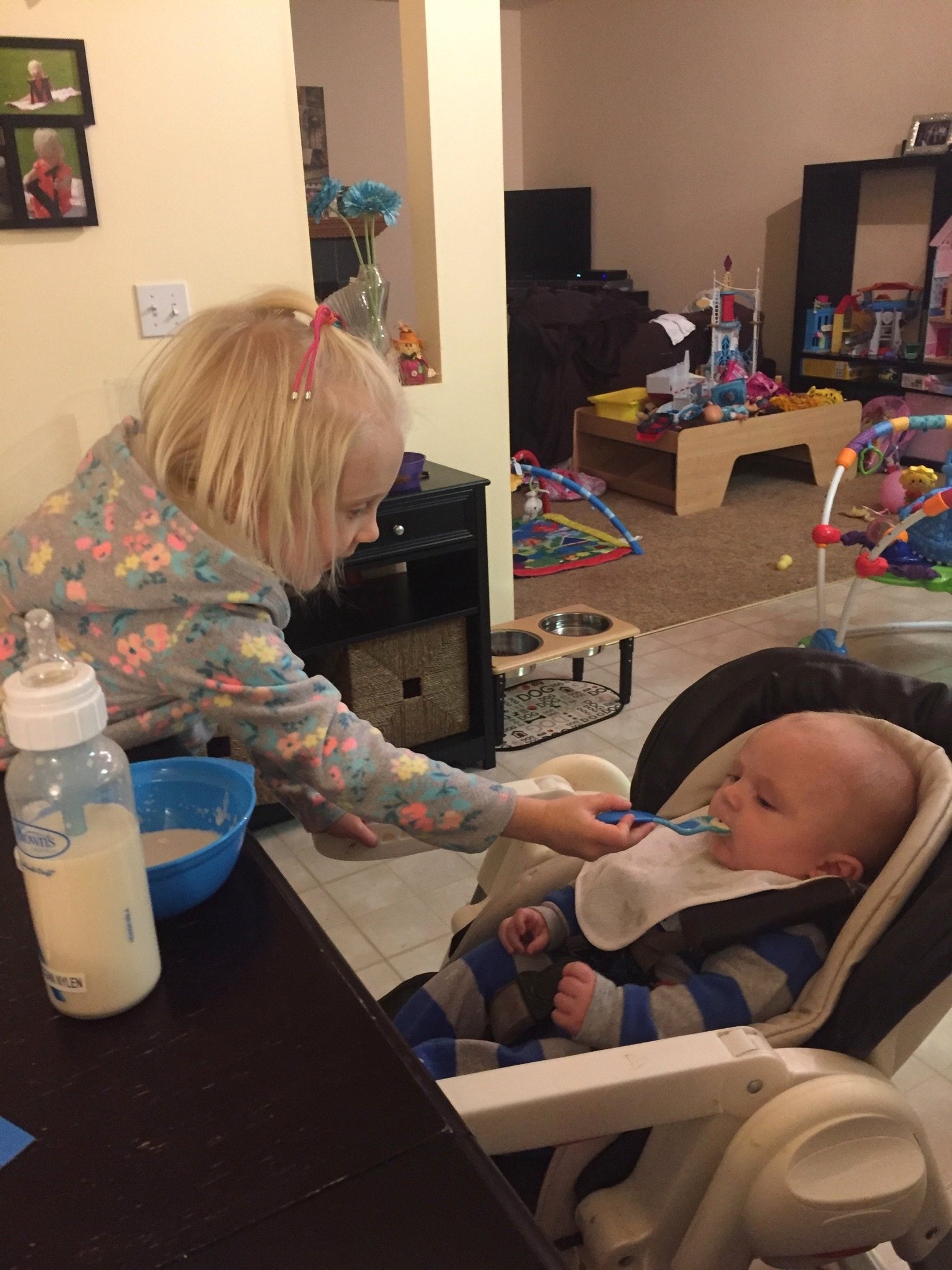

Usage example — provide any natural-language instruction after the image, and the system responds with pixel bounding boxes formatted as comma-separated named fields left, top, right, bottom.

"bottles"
left=0, top=607, right=165, bottom=1020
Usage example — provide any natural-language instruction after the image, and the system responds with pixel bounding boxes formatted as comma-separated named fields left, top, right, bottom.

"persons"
left=21, top=126, right=75, bottom=220
left=0, top=281, right=658, bottom=871
left=22, top=58, right=55, bottom=105
left=383, top=690, right=918, bottom=1130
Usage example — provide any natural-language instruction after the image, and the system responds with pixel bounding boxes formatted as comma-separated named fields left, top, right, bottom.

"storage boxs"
left=901, top=391, right=952, bottom=464
left=800, top=356, right=890, bottom=380
left=587, top=386, right=648, bottom=426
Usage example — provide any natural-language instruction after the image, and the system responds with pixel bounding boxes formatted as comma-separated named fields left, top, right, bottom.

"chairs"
left=371, top=638, right=951, bottom=1270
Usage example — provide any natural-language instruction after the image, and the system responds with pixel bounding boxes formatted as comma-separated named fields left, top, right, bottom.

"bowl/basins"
left=490, top=612, right=613, bottom=678
left=130, top=757, right=256, bottom=920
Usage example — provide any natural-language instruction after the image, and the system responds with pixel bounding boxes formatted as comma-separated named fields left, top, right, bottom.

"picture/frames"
left=903, top=111, right=952, bottom=155
left=0, top=122, right=98, bottom=230
left=0, top=35, right=96, bottom=127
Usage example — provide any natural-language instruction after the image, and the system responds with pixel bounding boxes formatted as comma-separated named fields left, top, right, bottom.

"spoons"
left=596, top=810, right=731, bottom=835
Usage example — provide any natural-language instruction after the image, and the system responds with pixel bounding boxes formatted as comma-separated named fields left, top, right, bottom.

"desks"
left=280, top=455, right=497, bottom=773
left=1, top=726, right=570, bottom=1270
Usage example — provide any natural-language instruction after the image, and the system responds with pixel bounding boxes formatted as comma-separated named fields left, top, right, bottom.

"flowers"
left=307, top=176, right=404, bottom=353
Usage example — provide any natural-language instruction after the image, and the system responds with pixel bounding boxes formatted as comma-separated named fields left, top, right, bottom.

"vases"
left=351, top=263, right=394, bottom=359
